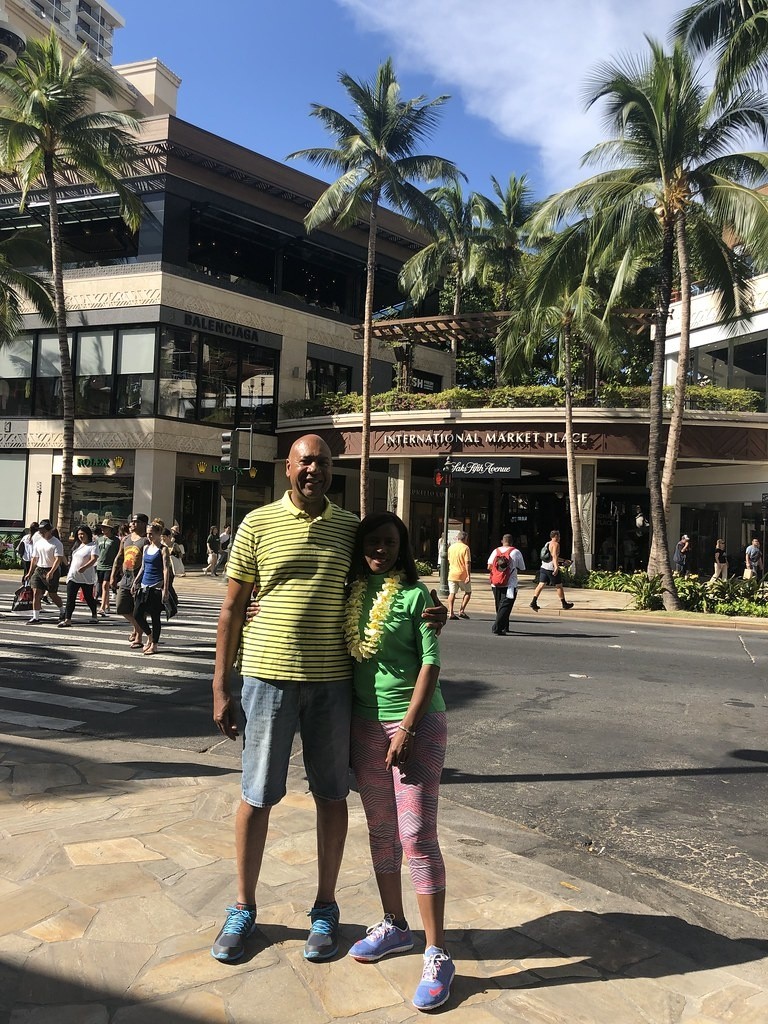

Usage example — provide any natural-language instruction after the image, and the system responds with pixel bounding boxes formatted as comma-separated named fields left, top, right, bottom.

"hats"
left=684, top=534, right=690, bottom=540
left=132, top=513, right=150, bottom=525
left=95, top=519, right=114, bottom=528
left=35, top=519, right=54, bottom=532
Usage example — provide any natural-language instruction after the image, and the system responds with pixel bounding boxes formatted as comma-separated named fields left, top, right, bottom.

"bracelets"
left=134, top=581, right=137, bottom=585
left=399, top=722, right=416, bottom=737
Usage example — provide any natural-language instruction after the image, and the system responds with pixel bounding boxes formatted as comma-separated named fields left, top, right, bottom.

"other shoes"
left=26, top=619, right=40, bottom=625
left=202, top=568, right=207, bottom=577
left=458, top=613, right=470, bottom=619
left=143, top=639, right=157, bottom=654
left=449, top=614, right=459, bottom=619
left=96, top=607, right=110, bottom=616
left=530, top=602, right=538, bottom=612
left=57, top=612, right=64, bottom=622
left=88, top=618, right=99, bottom=623
left=494, top=627, right=508, bottom=635
left=562, top=602, right=573, bottom=609
left=210, top=571, right=217, bottom=576
left=57, top=620, right=72, bottom=627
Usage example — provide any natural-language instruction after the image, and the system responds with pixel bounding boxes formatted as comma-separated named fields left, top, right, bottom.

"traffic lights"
left=220, top=431, right=239, bottom=467
left=434, top=470, right=452, bottom=488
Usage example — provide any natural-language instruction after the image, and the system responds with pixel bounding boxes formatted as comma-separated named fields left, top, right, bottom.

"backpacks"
left=490, top=546, right=516, bottom=586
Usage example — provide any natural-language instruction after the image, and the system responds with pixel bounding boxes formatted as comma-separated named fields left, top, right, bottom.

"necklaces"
left=342, top=568, right=407, bottom=663
left=73, top=542, right=90, bottom=554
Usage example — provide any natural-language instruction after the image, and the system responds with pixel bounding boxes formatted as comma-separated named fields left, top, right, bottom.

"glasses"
left=721, top=543, right=725, bottom=544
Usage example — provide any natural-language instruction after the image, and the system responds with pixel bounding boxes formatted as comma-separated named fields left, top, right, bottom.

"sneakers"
left=347, top=911, right=414, bottom=963
left=412, top=945, right=456, bottom=1010
left=304, top=903, right=341, bottom=959
left=210, top=900, right=257, bottom=962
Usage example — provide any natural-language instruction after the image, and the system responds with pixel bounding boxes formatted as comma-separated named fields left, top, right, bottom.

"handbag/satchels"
left=541, top=543, right=552, bottom=562
left=207, top=553, right=218, bottom=565
left=743, top=567, right=753, bottom=579
left=750, top=551, right=761, bottom=562
left=221, top=535, right=230, bottom=550
left=169, top=543, right=186, bottom=577
left=161, top=584, right=179, bottom=622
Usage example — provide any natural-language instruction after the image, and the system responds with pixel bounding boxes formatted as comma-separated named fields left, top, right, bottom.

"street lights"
left=36, top=482, right=43, bottom=523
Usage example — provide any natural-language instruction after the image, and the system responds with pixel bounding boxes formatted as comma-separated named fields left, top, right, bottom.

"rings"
left=399, top=761, right=405, bottom=764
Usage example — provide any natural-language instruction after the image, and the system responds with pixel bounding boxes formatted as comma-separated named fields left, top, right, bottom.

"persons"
left=202, top=525, right=233, bottom=578
left=110, top=513, right=171, bottom=649
left=620, top=533, right=636, bottom=570
left=673, top=535, right=690, bottom=578
left=710, top=539, right=727, bottom=582
left=242, top=511, right=455, bottom=1010
left=438, top=532, right=472, bottom=620
left=600, top=534, right=615, bottom=563
left=16, top=513, right=185, bottom=628
left=529, top=530, right=574, bottom=610
left=130, top=518, right=170, bottom=655
left=746, top=538, right=763, bottom=588
left=210, top=434, right=448, bottom=1010
left=487, top=534, right=525, bottom=634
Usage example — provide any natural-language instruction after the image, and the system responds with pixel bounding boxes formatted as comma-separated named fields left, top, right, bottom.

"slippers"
left=127, top=633, right=144, bottom=648
